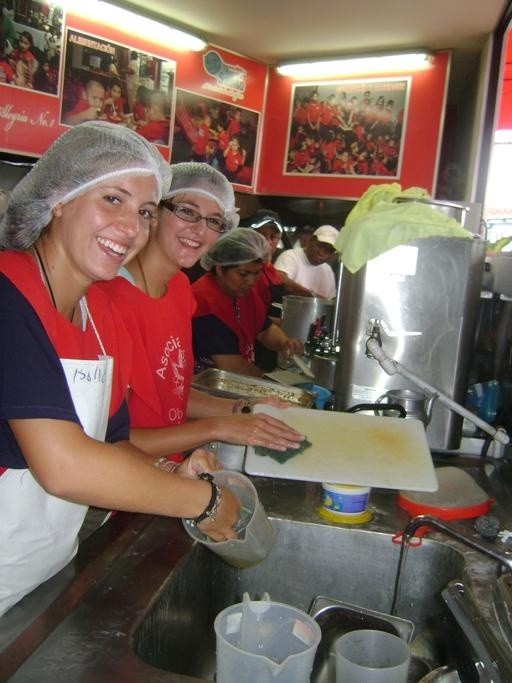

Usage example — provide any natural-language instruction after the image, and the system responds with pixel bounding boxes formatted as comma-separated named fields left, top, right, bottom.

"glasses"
left=159, top=197, right=230, bottom=234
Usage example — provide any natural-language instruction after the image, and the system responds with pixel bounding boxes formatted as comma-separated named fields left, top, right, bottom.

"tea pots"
left=372, top=386, right=441, bottom=429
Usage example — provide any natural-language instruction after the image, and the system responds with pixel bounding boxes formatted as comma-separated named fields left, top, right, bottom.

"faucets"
left=390, top=514, right=511, bottom=617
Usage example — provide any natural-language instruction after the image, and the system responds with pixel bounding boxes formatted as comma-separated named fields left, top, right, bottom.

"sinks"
left=130, top=513, right=498, bottom=683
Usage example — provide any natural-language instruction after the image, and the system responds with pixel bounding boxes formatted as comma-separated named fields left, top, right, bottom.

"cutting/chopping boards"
left=241, top=400, right=440, bottom=495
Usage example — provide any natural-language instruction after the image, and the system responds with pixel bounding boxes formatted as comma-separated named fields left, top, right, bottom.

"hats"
left=248, top=208, right=283, bottom=233
left=313, top=224, right=341, bottom=250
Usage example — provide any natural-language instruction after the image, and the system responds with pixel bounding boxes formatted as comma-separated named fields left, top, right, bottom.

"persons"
left=287, top=85, right=404, bottom=175
left=0, top=0, right=63, bottom=95
left=176, top=98, right=249, bottom=184
left=63, top=36, right=175, bottom=149
